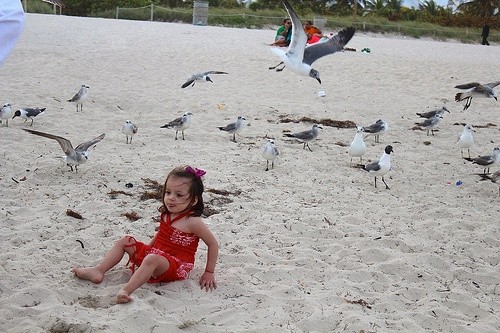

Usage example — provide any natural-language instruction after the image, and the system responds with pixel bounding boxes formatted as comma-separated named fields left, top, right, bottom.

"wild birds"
left=408, top=114, right=443, bottom=136
left=361, top=119, right=388, bottom=144
left=262, top=138, right=279, bottom=171
left=268, top=0, right=356, bottom=84
left=348, top=126, right=366, bottom=168
left=455, top=81, right=500, bottom=111
left=415, top=106, right=451, bottom=132
left=479, top=169, right=500, bottom=196
left=180, top=70, right=229, bottom=89
left=21, top=127, right=106, bottom=175
left=12, top=106, right=46, bottom=127
left=281, top=125, right=324, bottom=152
left=216, top=116, right=246, bottom=143
left=0, top=103, right=12, bottom=128
left=463, top=147, right=500, bottom=175
left=66, top=84, right=90, bottom=112
left=351, top=145, right=394, bottom=190
left=122, top=120, right=138, bottom=144
left=455, top=124, right=477, bottom=159
left=159, top=112, right=194, bottom=141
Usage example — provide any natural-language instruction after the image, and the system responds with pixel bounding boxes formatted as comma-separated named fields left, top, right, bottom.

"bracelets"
left=205, top=269, right=214, bottom=274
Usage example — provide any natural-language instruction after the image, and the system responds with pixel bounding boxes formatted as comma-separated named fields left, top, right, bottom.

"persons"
left=72, top=164, right=219, bottom=304
left=305, top=32, right=333, bottom=45
left=270, top=18, right=292, bottom=47
left=303, top=20, right=322, bottom=37
left=480, top=21, right=491, bottom=47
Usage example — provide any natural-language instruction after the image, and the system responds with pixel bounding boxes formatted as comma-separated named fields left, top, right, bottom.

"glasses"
left=286, top=22, right=291, bottom=25
